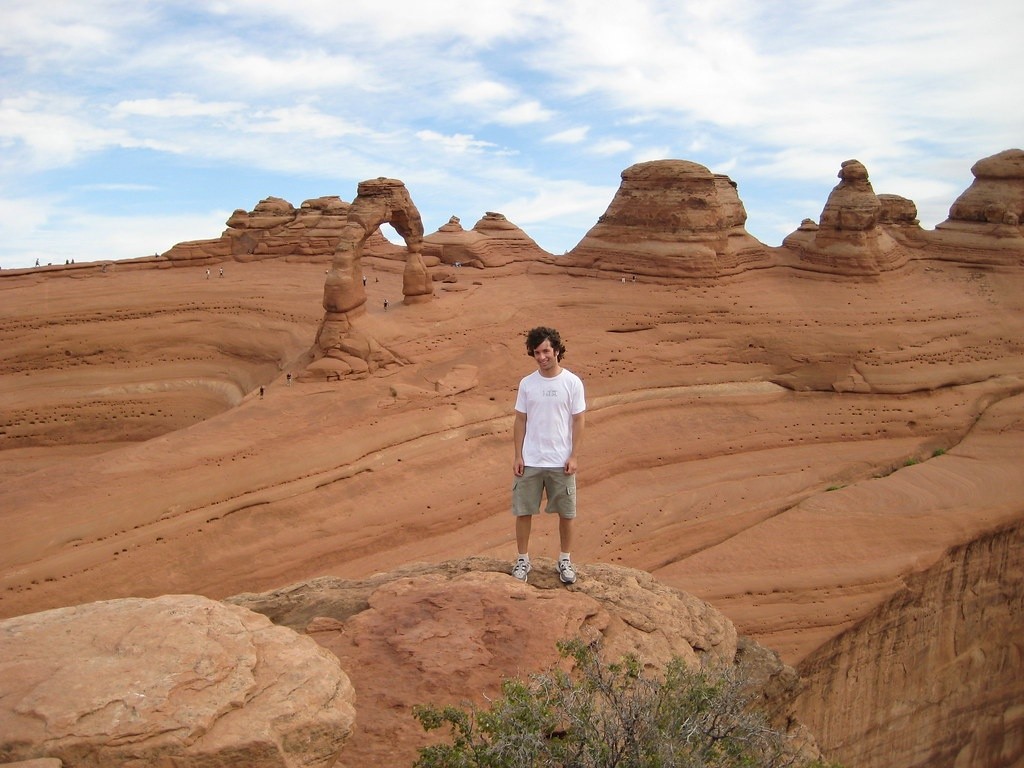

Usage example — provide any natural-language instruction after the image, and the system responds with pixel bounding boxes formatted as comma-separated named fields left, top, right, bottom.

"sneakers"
left=512, top=558, right=531, bottom=582
left=556, top=559, right=579, bottom=583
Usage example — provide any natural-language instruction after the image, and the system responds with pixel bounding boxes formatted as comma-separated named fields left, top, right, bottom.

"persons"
left=35, top=258, right=41, bottom=267
left=206, top=268, right=211, bottom=280
left=383, top=298, right=389, bottom=312
left=622, top=274, right=625, bottom=284
left=258, top=386, right=264, bottom=400
left=219, top=266, right=224, bottom=277
left=451, top=258, right=461, bottom=268
left=513, top=326, right=586, bottom=585
left=285, top=373, right=292, bottom=386
left=363, top=275, right=367, bottom=287
left=631, top=275, right=638, bottom=286
